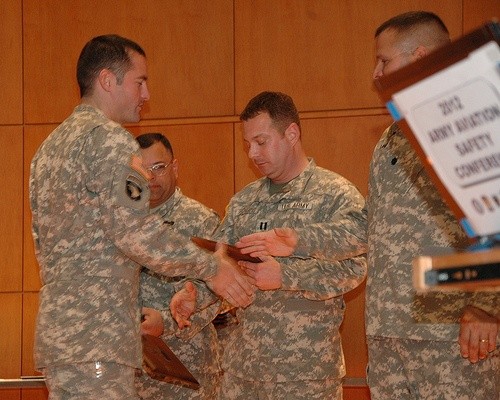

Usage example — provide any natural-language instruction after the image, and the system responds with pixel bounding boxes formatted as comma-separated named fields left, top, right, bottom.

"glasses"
left=143, top=162, right=171, bottom=177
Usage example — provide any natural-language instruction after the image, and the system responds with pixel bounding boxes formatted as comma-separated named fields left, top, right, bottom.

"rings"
left=479, top=338, right=490, bottom=343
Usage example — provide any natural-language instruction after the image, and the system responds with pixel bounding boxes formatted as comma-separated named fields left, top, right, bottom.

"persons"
left=133, top=130, right=229, bottom=399
left=170, top=90, right=367, bottom=400
left=234, top=7, right=500, bottom=400
left=28, top=35, right=252, bottom=399
